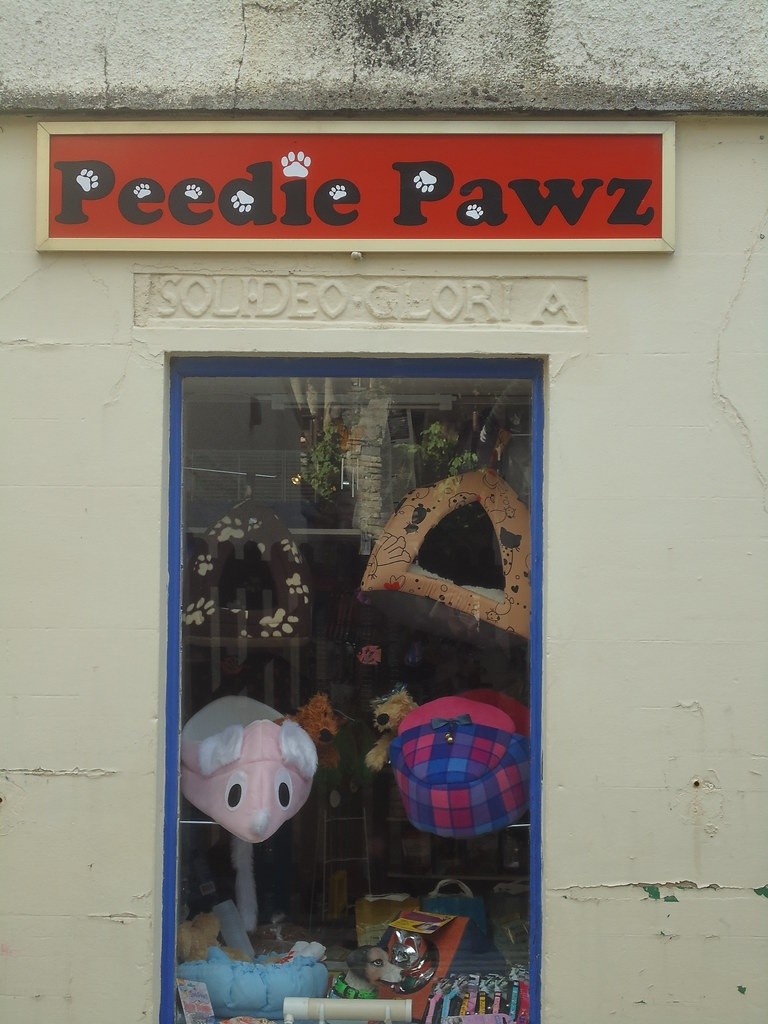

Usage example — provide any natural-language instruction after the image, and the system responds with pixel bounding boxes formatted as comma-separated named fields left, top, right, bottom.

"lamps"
left=271, top=393, right=458, bottom=411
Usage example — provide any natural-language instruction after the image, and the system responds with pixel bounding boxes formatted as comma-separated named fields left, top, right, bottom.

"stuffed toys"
left=364, top=690, right=420, bottom=771
left=181, top=694, right=318, bottom=936
left=274, top=689, right=347, bottom=769
left=177, top=912, right=284, bottom=964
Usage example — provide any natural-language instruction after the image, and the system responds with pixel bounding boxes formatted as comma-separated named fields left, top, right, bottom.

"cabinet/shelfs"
left=386, top=794, right=531, bottom=935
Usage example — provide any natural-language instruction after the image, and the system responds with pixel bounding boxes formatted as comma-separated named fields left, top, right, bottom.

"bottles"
left=210, top=896, right=255, bottom=958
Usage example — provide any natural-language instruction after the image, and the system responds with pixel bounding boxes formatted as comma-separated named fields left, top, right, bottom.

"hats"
left=390, top=687, right=532, bottom=843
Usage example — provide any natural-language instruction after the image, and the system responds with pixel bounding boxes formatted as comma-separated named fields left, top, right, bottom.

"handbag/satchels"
left=422, top=880, right=494, bottom=973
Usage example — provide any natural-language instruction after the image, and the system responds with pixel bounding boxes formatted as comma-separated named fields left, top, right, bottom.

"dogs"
left=328, top=946, right=407, bottom=999
